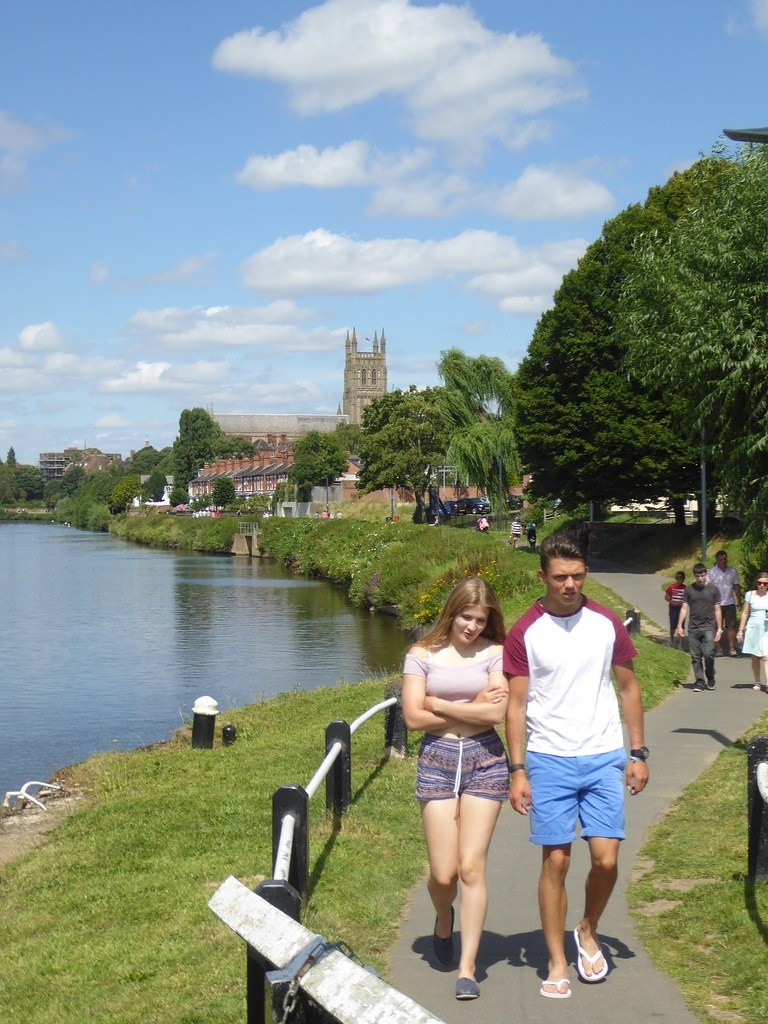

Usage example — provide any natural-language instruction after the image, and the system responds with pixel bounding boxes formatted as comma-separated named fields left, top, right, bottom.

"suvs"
left=425, top=497, right=490, bottom=518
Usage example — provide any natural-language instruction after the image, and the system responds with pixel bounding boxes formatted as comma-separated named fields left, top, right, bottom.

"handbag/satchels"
left=509, top=535, right=512, bottom=545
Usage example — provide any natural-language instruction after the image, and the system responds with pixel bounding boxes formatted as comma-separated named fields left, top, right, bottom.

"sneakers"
left=694, top=680, right=704, bottom=691
left=707, top=677, right=715, bottom=689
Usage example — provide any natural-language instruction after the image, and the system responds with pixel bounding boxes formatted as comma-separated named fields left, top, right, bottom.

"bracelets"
left=508, top=763, right=525, bottom=772
left=718, top=629, right=723, bottom=633
left=738, top=628, right=744, bottom=630
left=629, top=756, right=639, bottom=761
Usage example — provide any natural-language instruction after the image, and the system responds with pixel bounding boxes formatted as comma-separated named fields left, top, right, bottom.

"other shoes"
left=433, top=905, right=454, bottom=966
left=753, top=682, right=761, bottom=691
left=456, top=978, right=479, bottom=998
left=716, top=649, right=723, bottom=657
left=729, top=650, right=737, bottom=656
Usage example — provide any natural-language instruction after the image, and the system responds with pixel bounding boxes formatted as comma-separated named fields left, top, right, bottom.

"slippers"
left=539, top=979, right=572, bottom=998
left=574, top=927, right=609, bottom=981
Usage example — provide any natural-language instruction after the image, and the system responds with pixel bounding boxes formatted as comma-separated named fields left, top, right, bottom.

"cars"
left=505, top=494, right=521, bottom=509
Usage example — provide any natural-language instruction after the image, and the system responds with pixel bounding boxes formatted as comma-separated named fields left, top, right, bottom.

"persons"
left=510, top=516, right=522, bottom=547
left=527, top=527, right=537, bottom=546
left=736, top=572, right=768, bottom=691
left=499, top=535, right=649, bottom=999
left=192, top=509, right=343, bottom=520
left=706, top=551, right=742, bottom=656
left=402, top=577, right=509, bottom=999
left=477, top=514, right=489, bottom=532
left=665, top=571, right=687, bottom=649
left=677, top=564, right=721, bottom=692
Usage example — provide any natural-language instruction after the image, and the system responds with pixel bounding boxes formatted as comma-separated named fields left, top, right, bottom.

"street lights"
left=322, top=477, right=328, bottom=516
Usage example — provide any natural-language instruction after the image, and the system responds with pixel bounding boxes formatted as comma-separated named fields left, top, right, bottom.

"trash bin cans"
left=386, top=517, right=391, bottom=522
left=394, top=516, right=399, bottom=521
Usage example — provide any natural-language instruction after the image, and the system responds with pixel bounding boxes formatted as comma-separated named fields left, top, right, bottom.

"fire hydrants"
left=191, top=696, right=218, bottom=749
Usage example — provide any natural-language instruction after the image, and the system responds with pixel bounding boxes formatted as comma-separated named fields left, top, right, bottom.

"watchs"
left=630, top=746, right=649, bottom=760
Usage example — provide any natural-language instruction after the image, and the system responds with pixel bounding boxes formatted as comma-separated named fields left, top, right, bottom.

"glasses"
left=756, top=581, right=768, bottom=586
